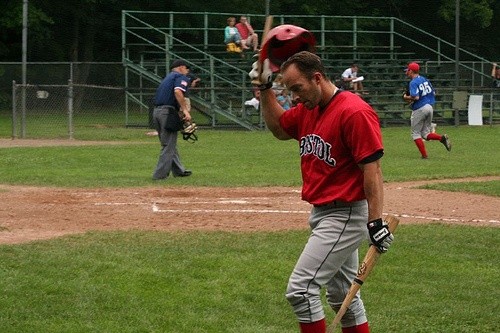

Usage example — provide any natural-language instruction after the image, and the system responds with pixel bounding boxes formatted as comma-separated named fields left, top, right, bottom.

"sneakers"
left=440, top=134, right=451, bottom=151
left=184, top=170, right=192, bottom=176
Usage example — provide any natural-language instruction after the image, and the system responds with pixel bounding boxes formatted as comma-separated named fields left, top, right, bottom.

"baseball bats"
left=326, top=213, right=400, bottom=333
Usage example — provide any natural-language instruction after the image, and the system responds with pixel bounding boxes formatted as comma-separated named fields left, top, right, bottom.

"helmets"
left=260, top=24, right=314, bottom=73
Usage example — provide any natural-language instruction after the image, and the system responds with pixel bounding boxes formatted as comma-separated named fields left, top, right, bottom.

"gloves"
left=367, top=217, right=394, bottom=253
left=249, top=54, right=274, bottom=92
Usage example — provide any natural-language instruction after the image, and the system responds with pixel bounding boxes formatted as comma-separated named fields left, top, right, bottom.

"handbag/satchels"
left=164, top=108, right=181, bottom=132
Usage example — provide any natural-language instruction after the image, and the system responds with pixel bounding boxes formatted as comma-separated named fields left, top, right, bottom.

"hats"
left=404, top=62, right=419, bottom=72
left=171, top=59, right=191, bottom=69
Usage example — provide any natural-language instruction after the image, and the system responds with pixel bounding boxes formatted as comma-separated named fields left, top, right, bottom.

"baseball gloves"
left=180, top=114, right=198, bottom=143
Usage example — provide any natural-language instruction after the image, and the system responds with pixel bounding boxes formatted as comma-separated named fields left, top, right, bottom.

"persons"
left=235, top=15, right=262, bottom=53
left=249, top=54, right=260, bottom=100
left=402, top=61, right=451, bottom=159
left=341, top=63, right=365, bottom=95
left=491, top=62, right=500, bottom=88
left=151, top=60, right=201, bottom=179
left=224, top=16, right=250, bottom=56
left=247, top=51, right=395, bottom=332
left=277, top=89, right=293, bottom=110
